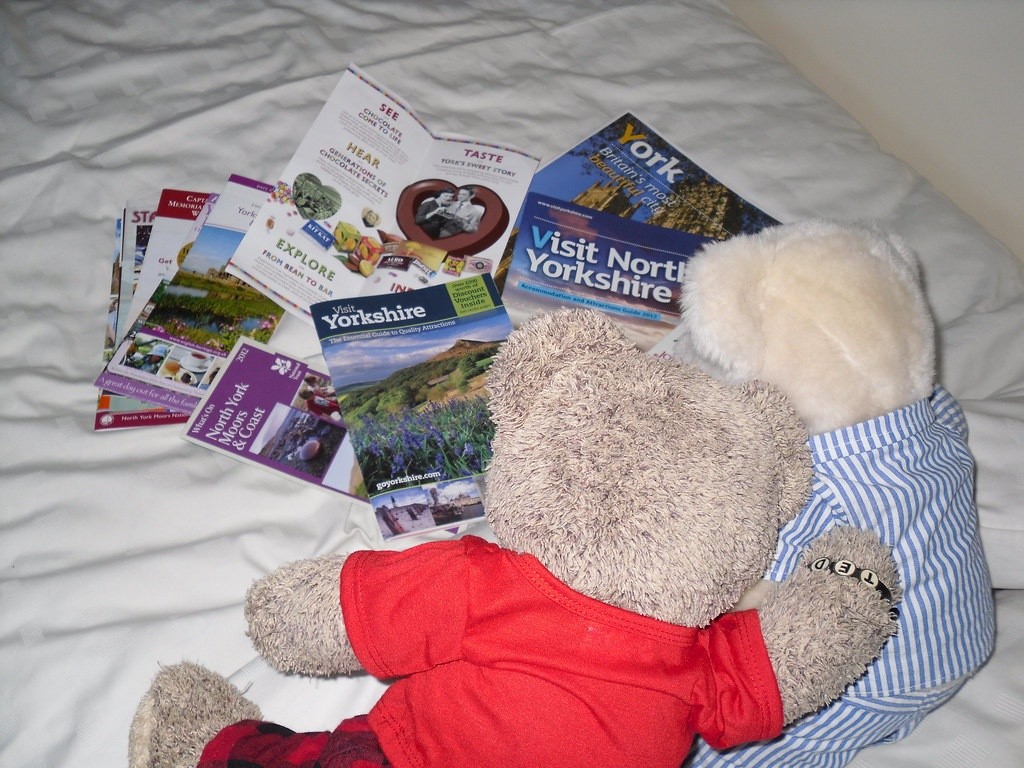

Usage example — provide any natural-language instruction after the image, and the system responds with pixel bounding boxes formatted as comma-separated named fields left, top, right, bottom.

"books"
left=89, top=60, right=790, bottom=550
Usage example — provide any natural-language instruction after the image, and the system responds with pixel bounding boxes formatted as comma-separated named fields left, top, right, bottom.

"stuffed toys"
left=127, top=305, right=901, bottom=767
left=673, top=216, right=996, bottom=765
